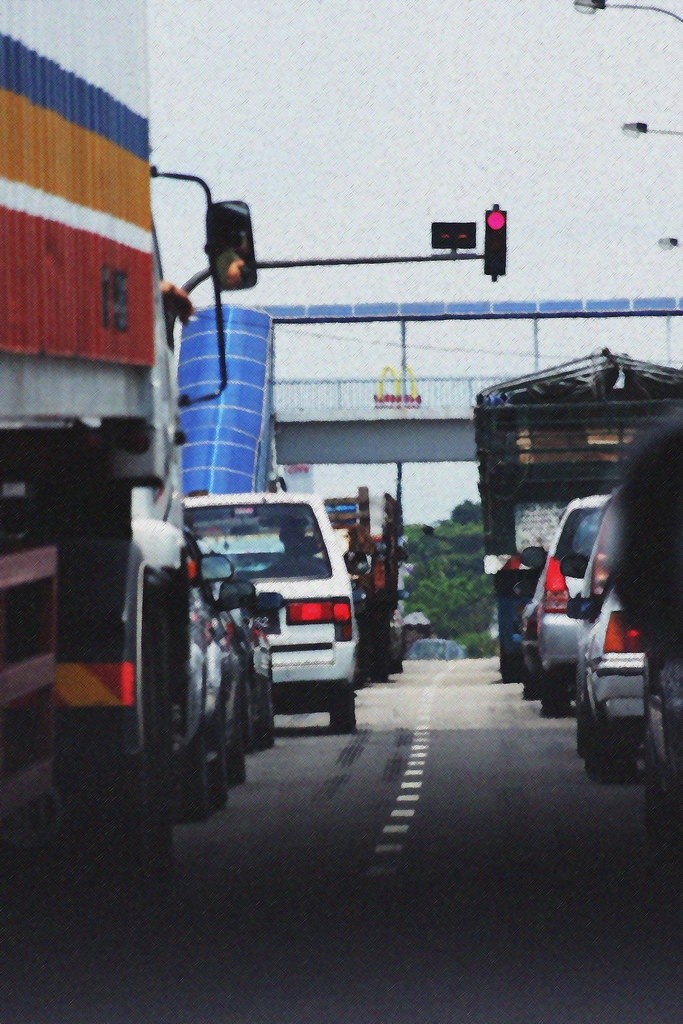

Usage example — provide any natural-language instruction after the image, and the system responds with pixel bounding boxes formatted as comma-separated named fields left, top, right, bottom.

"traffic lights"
left=482, top=205, right=508, bottom=280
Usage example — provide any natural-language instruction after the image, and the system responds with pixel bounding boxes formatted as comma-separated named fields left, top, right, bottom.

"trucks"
left=471, top=351, right=682, bottom=684
left=322, top=489, right=405, bottom=688
left=0, top=35, right=259, bottom=822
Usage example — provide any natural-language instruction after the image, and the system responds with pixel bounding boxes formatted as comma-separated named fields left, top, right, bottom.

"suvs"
left=566, top=486, right=649, bottom=778
left=178, top=480, right=370, bottom=731
left=509, top=495, right=609, bottom=715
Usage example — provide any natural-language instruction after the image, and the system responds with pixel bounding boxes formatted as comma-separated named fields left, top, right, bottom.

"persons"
left=259, top=520, right=331, bottom=576
